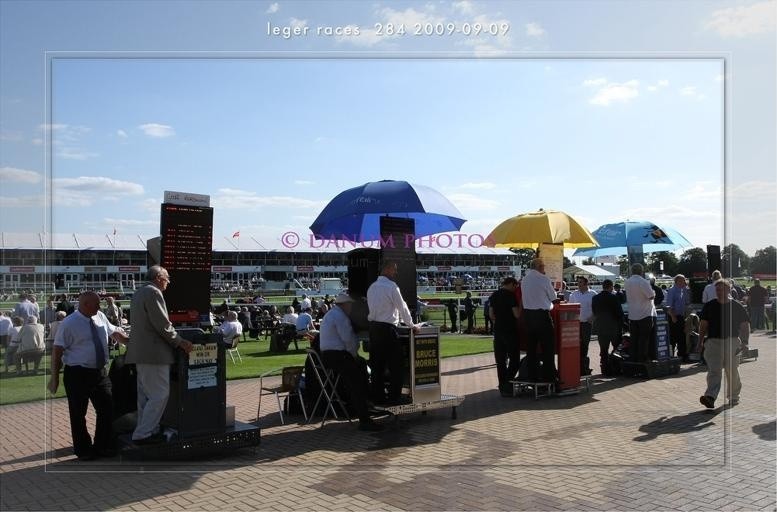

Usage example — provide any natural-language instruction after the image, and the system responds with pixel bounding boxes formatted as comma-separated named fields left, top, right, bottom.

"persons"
left=520, top=257, right=558, bottom=382
left=486, top=276, right=523, bottom=397
left=1, top=280, right=137, bottom=384
left=412, top=262, right=776, bottom=394
left=694, top=278, right=750, bottom=408
left=210, top=272, right=348, bottom=360
left=45, top=291, right=131, bottom=463
left=367, top=261, right=421, bottom=407
left=123, top=264, right=194, bottom=447
left=319, top=293, right=386, bottom=431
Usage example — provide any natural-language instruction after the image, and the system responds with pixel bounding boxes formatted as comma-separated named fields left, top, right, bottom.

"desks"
left=250, top=319, right=272, bottom=340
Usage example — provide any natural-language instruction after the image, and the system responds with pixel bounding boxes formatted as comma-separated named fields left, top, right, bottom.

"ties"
left=89, top=318, right=105, bottom=370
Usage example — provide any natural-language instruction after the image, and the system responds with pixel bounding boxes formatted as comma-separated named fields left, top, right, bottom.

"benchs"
left=243, top=328, right=279, bottom=341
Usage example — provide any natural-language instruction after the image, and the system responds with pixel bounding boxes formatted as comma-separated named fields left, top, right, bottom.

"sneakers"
left=700, top=396, right=714, bottom=408
left=729, top=399, right=739, bottom=405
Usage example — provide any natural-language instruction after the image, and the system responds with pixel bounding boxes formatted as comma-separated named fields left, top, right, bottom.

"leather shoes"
left=134, top=433, right=167, bottom=446
left=359, top=421, right=385, bottom=432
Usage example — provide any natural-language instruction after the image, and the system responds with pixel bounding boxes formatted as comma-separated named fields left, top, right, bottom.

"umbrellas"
left=309, top=179, right=468, bottom=242
left=571, top=219, right=695, bottom=276
left=481, top=208, right=600, bottom=253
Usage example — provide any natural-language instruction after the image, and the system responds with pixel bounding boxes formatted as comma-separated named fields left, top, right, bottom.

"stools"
left=22, top=352, right=42, bottom=376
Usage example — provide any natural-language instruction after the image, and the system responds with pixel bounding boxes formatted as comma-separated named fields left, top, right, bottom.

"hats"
left=335, top=296, right=354, bottom=303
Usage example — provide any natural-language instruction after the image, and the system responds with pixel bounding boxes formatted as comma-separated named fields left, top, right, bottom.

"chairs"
left=225, top=335, right=243, bottom=365
left=257, top=347, right=353, bottom=431
left=281, top=322, right=300, bottom=351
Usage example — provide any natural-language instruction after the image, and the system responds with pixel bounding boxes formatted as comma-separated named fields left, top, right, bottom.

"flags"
left=233, top=232, right=240, bottom=239
left=113, top=229, right=117, bottom=235
